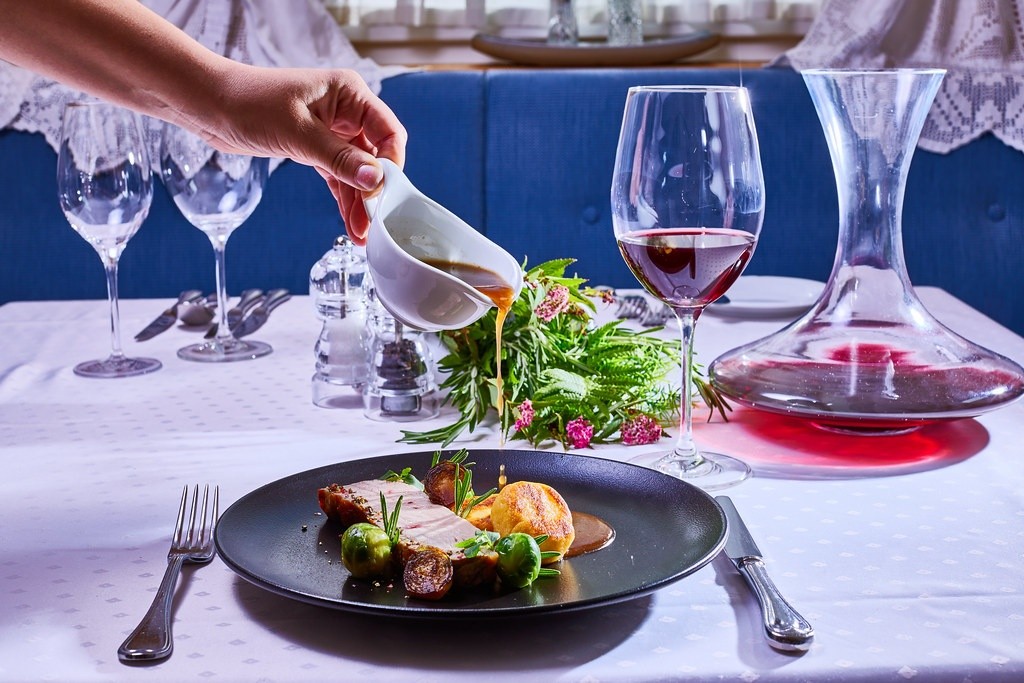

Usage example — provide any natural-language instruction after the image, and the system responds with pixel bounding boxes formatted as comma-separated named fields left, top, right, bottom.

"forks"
left=115, top=482, right=220, bottom=666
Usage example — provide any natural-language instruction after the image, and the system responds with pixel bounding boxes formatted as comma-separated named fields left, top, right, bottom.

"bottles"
left=308, top=235, right=376, bottom=411
left=361, top=260, right=443, bottom=424
left=707, top=61, right=1024, bottom=440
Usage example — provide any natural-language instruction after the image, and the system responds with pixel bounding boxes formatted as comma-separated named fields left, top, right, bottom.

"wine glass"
left=155, top=111, right=274, bottom=366
left=611, top=82, right=768, bottom=488
left=56, top=100, right=167, bottom=384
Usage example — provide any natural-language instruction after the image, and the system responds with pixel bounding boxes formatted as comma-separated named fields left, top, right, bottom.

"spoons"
left=176, top=292, right=224, bottom=324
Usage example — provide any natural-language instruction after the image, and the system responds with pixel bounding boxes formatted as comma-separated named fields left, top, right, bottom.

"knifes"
left=711, top=495, right=816, bottom=653
left=135, top=288, right=200, bottom=343
left=233, top=288, right=292, bottom=341
left=207, top=286, right=264, bottom=339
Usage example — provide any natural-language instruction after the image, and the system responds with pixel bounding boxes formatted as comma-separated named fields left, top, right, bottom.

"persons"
left=0, top=0, right=409, bottom=243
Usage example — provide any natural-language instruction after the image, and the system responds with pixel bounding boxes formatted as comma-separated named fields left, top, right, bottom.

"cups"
left=361, top=155, right=525, bottom=333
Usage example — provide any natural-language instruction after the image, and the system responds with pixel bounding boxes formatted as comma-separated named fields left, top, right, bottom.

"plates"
left=214, top=445, right=731, bottom=640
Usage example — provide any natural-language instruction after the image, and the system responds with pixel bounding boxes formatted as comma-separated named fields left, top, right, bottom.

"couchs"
left=1, top=67, right=1024, bottom=338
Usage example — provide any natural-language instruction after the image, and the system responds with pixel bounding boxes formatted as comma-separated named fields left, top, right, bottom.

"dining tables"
left=1, top=284, right=1023, bottom=683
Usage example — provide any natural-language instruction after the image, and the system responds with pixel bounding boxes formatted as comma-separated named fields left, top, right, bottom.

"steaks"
left=318, top=478, right=494, bottom=580
